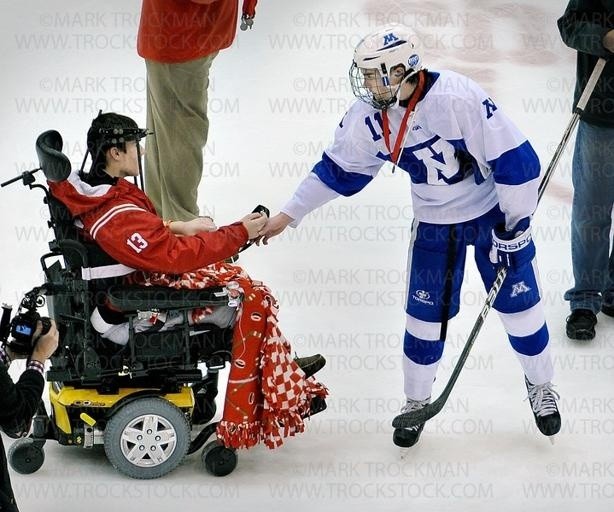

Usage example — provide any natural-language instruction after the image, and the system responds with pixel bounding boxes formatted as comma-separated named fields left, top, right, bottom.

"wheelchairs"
left=1, top=130, right=327, bottom=480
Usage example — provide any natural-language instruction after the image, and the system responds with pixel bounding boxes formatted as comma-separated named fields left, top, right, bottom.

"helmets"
left=352, top=22, right=427, bottom=91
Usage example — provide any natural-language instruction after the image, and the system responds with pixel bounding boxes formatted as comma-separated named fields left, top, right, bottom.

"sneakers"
left=566, top=308, right=598, bottom=340
left=291, top=353, right=327, bottom=381
left=393, top=397, right=429, bottom=448
left=524, top=373, right=562, bottom=437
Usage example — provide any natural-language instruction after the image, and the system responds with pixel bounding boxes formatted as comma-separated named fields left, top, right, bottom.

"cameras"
left=10, top=313, right=66, bottom=352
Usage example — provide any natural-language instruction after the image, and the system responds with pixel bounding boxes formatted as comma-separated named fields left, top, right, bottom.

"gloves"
left=487, top=224, right=538, bottom=283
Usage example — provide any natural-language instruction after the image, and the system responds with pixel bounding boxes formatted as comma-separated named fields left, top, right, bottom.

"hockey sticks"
left=392, top=58, right=606, bottom=429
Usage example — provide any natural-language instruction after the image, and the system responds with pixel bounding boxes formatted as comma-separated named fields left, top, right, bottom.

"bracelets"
left=165, top=220, right=173, bottom=229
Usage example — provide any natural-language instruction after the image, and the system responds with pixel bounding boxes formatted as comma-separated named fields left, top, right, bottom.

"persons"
left=1, top=318, right=61, bottom=511
left=46, top=110, right=330, bottom=452
left=248, top=20, right=563, bottom=448
left=135, top=1, right=258, bottom=223
left=556, top=1, right=614, bottom=341
